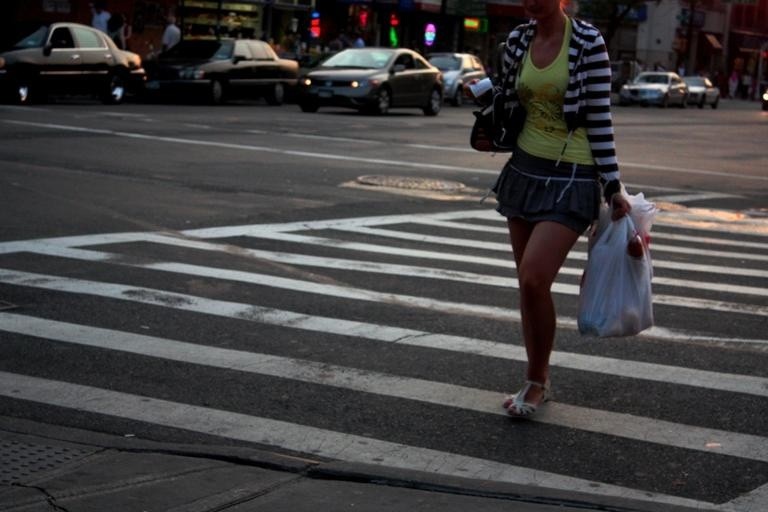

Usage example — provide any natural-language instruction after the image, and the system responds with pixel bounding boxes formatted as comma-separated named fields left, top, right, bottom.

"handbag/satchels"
left=470, top=41, right=528, bottom=154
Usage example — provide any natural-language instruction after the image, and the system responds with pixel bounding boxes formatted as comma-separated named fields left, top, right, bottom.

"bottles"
left=626, top=230, right=645, bottom=259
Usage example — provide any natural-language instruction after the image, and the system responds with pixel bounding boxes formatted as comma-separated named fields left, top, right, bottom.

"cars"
left=610, top=60, right=642, bottom=89
left=620, top=72, right=688, bottom=108
left=683, top=76, right=720, bottom=108
left=295, top=47, right=444, bottom=118
left=761, top=89, right=767, bottom=109
left=1, top=22, right=148, bottom=105
left=425, top=51, right=486, bottom=106
left=142, top=37, right=300, bottom=108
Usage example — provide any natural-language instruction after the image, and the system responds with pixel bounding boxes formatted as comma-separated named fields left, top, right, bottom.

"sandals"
left=502, top=378, right=553, bottom=417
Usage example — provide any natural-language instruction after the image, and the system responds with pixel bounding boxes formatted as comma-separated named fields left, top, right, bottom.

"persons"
left=637, top=59, right=752, bottom=100
left=464, top=0, right=634, bottom=417
left=89, top=0, right=366, bottom=62
